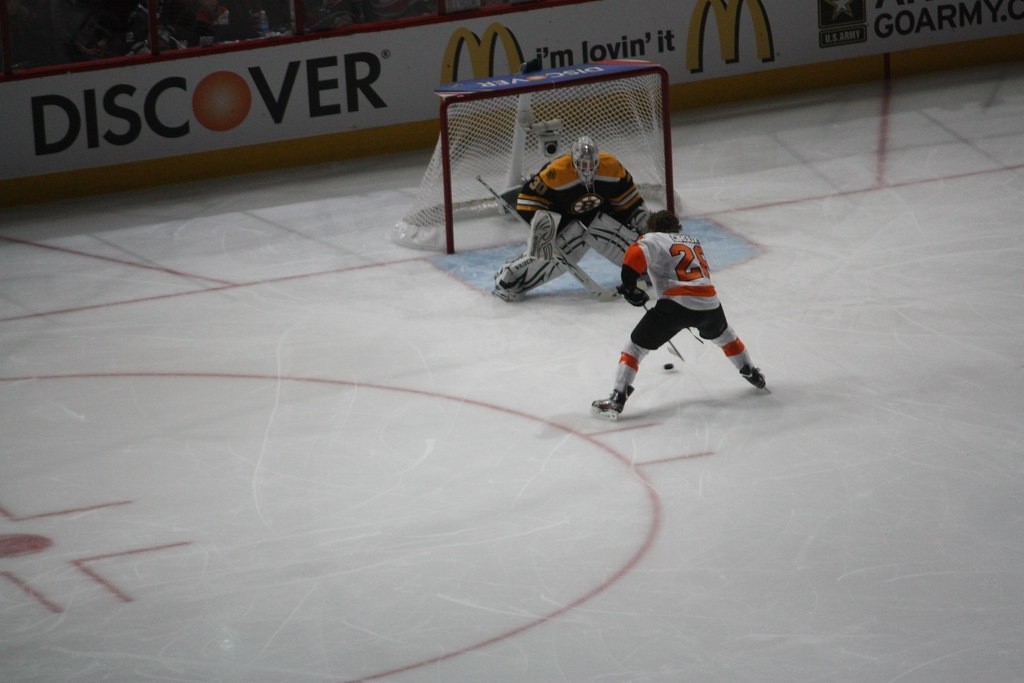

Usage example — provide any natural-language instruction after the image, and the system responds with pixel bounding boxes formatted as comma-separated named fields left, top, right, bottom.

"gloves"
left=616, top=285, right=650, bottom=306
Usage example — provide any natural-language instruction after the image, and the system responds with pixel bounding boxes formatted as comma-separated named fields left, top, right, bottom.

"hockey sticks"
left=642, top=303, right=686, bottom=363
left=475, top=175, right=623, bottom=302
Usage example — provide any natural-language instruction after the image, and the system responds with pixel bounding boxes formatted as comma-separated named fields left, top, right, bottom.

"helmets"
left=571, top=136, right=600, bottom=183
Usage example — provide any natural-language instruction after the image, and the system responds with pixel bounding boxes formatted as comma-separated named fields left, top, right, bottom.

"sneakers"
left=592, top=386, right=634, bottom=422
left=740, top=365, right=771, bottom=393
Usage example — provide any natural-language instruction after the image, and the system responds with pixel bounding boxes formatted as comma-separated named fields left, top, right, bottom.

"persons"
left=7, top=0, right=30, bottom=28
left=592, top=209, right=772, bottom=422
left=67, top=0, right=439, bottom=63
left=493, top=137, right=650, bottom=303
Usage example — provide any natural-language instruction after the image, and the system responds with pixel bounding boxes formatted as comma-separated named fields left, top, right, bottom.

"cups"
left=200, top=36, right=212, bottom=47
left=178, top=40, right=187, bottom=49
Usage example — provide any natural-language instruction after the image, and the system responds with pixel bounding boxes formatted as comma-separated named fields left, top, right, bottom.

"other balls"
left=664, top=363, right=673, bottom=370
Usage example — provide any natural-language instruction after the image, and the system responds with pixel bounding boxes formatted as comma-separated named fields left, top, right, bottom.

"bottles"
left=257, top=10, right=269, bottom=36
left=144, top=40, right=151, bottom=54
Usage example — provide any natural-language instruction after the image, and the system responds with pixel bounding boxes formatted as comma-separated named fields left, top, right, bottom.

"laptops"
left=213, top=23, right=243, bottom=43
